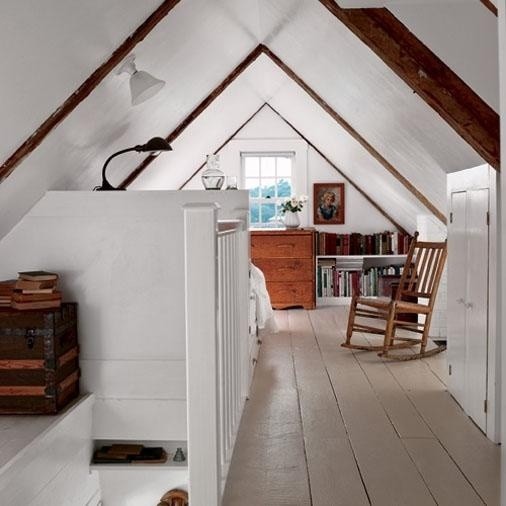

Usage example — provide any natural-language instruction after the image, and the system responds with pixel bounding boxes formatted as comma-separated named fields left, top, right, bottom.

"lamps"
left=93, top=137, right=173, bottom=190
left=113, top=54, right=165, bottom=107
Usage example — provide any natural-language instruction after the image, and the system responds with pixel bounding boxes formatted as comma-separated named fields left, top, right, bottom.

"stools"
left=378, top=275, right=418, bottom=326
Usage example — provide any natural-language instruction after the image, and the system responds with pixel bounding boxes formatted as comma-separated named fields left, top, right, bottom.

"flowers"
left=276, top=192, right=310, bottom=213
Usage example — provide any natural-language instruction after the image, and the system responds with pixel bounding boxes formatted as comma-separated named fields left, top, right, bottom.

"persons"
left=316, top=190, right=338, bottom=220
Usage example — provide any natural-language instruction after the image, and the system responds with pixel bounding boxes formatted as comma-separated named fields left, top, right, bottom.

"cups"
left=227, top=175, right=237, bottom=190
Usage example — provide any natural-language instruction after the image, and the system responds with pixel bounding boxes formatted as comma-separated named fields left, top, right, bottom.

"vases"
left=283, top=210, right=300, bottom=228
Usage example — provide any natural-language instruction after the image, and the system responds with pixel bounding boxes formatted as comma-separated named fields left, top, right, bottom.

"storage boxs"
left=0, top=302, right=81, bottom=416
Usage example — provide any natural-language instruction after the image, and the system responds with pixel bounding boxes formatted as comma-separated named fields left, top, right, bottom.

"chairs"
left=341, top=230, right=447, bottom=361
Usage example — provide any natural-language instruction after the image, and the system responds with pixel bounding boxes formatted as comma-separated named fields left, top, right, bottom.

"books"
left=90, top=442, right=166, bottom=463
left=315, top=230, right=415, bottom=297
left=0, top=269, right=62, bottom=311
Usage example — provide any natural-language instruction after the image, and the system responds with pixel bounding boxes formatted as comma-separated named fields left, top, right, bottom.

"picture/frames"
left=313, top=183, right=345, bottom=225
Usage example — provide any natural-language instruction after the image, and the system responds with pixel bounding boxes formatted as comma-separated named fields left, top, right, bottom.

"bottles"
left=201, top=155, right=225, bottom=190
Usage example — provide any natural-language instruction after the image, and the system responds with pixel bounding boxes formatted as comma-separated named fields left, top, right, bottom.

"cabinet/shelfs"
left=250, top=226, right=317, bottom=310
left=0, top=392, right=102, bottom=506
left=315, top=254, right=418, bottom=307
left=446, top=163, right=501, bottom=445
